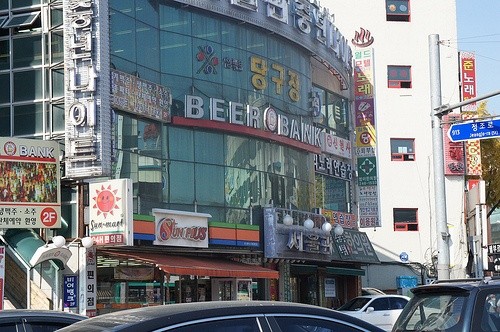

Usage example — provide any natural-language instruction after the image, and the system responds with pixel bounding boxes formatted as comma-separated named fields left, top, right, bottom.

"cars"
left=362, top=287, right=386, bottom=295
left=50, top=300, right=386, bottom=332
left=334, top=295, right=443, bottom=332
left=0, top=310, right=90, bottom=332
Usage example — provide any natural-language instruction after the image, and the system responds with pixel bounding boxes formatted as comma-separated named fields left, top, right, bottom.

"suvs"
left=391, top=278, right=500, bottom=332
